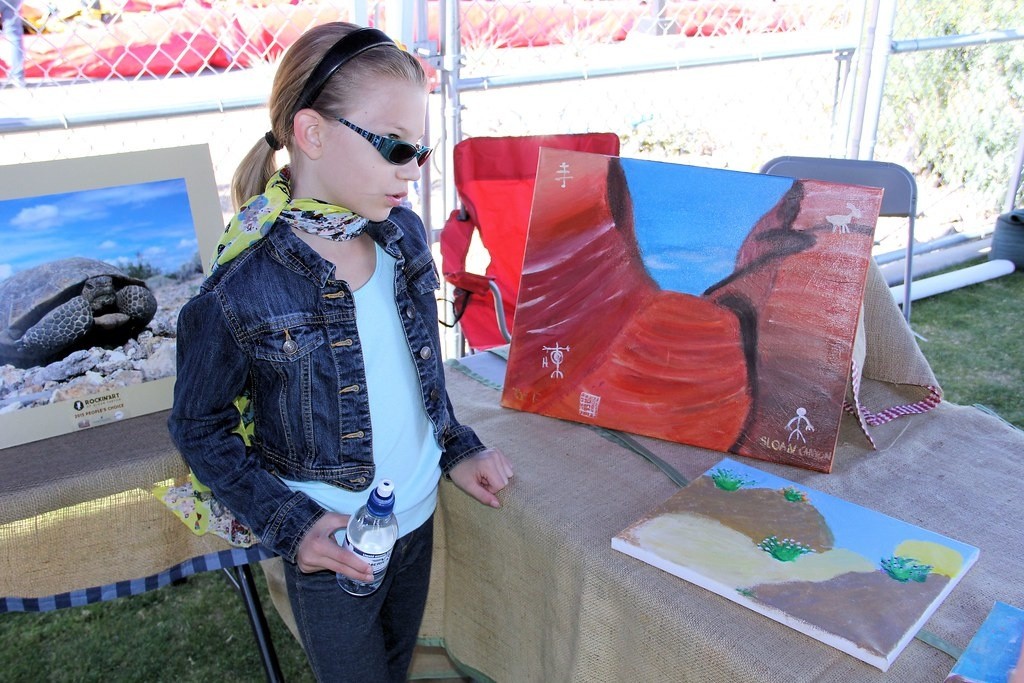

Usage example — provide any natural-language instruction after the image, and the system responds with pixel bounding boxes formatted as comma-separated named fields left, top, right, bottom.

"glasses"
left=315, top=105, right=431, bottom=167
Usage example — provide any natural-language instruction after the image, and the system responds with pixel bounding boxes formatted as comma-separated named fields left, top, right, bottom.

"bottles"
left=336, top=479, right=399, bottom=596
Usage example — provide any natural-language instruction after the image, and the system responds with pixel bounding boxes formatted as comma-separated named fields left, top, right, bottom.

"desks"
left=0, top=404, right=289, bottom=683
left=434, top=341, right=1022, bottom=683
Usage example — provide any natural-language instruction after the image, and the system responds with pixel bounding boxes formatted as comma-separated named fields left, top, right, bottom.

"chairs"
left=758, top=158, right=916, bottom=328
left=440, top=131, right=620, bottom=353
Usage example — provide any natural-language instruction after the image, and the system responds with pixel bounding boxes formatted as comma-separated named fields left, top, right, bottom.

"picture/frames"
left=0, top=144, right=224, bottom=450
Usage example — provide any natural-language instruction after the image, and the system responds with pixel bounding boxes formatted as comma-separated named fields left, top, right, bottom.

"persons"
left=166, top=19, right=515, bottom=683
